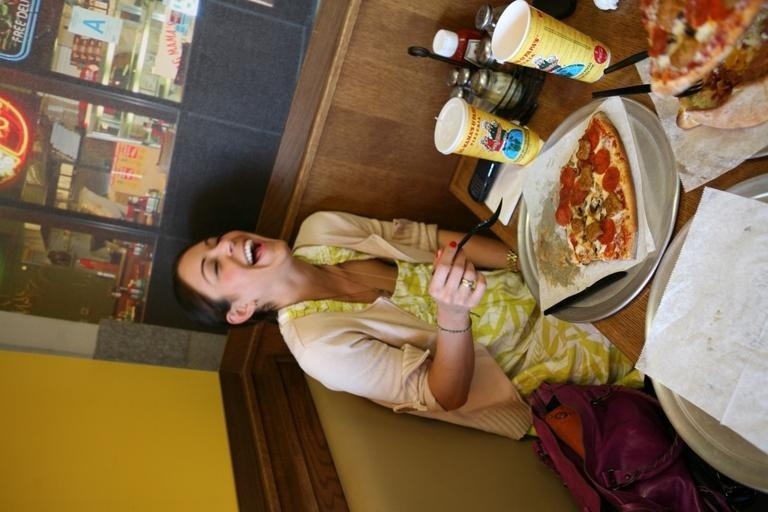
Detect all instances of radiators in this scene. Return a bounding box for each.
[450,1,767,371]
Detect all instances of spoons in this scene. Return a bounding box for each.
[431,196,504,261]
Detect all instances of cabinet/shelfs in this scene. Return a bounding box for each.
[85,0,173,147]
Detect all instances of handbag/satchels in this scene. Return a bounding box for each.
[522,376,733,511]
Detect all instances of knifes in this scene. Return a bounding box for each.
[543,271,629,316]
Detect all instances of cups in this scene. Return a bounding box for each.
[490,1,612,84]
[433,96,546,167]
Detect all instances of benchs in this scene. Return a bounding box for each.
[305,375,583,511]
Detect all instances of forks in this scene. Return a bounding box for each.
[591,77,704,100]
